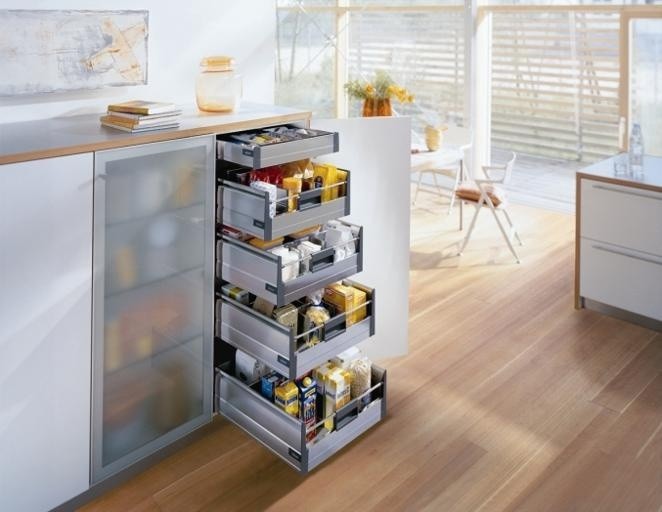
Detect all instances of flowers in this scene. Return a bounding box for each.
[342,68,415,104]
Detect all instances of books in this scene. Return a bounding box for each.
[98,99,183,134]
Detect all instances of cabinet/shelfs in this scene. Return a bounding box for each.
[573,151,662,333]
[1,98,411,512]
[362,99,393,117]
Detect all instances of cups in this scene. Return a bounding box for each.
[613,154,628,175]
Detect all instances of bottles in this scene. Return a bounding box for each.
[193,54,245,113]
[628,122,645,174]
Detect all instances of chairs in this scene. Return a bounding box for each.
[411,128,524,264]
